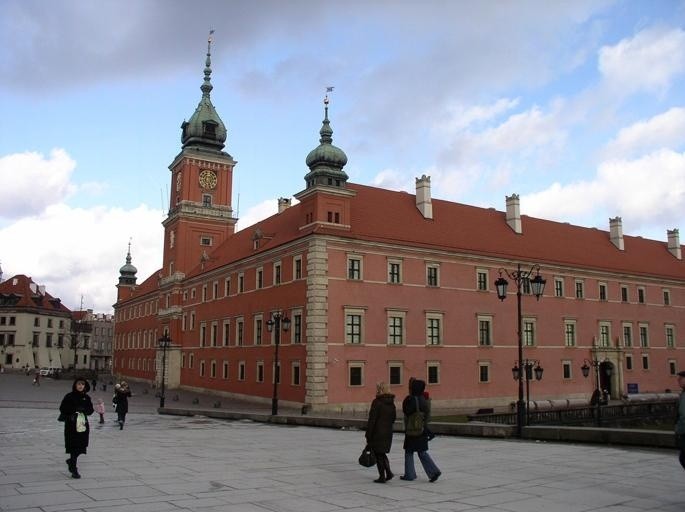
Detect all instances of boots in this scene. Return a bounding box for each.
[372,471,386,483]
[386,466,396,480]
[118,420,124,430]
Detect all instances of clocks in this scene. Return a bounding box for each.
[198,168,220,190]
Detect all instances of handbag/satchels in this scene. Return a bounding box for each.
[57,412,66,422]
[358,446,378,468]
[406,410,425,435]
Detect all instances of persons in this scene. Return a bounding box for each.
[57,377,93,479]
[92,379,96,391]
[112,383,122,407]
[96,398,105,423]
[408,376,436,440]
[400,379,441,482]
[112,379,131,430]
[677,370,685,469]
[366,380,395,483]
[31,372,39,387]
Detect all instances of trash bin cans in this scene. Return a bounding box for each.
[302,405,307,414]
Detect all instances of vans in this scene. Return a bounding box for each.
[39,366,62,377]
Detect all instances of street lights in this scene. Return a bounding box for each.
[158,327,173,408]
[510,358,544,426]
[265,306,291,415]
[494,263,549,426]
[582,355,613,425]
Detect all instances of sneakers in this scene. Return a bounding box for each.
[399,474,413,480]
[429,470,441,482]
[71,468,81,478]
[65,457,72,474]
